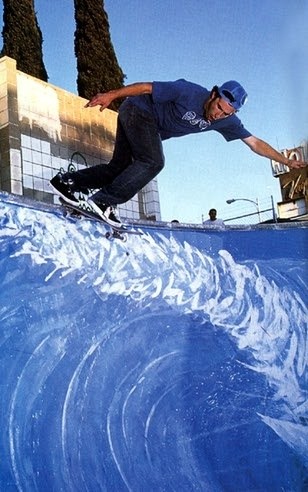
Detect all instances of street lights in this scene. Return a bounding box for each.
[226,198,260,224]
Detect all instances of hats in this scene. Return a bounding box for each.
[217,80,249,113]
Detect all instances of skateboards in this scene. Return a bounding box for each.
[59,197,143,242]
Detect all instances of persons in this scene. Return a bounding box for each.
[48,78,307,231]
[203,208,226,229]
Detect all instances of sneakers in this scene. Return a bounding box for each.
[87,198,122,229]
[46,172,81,207]
